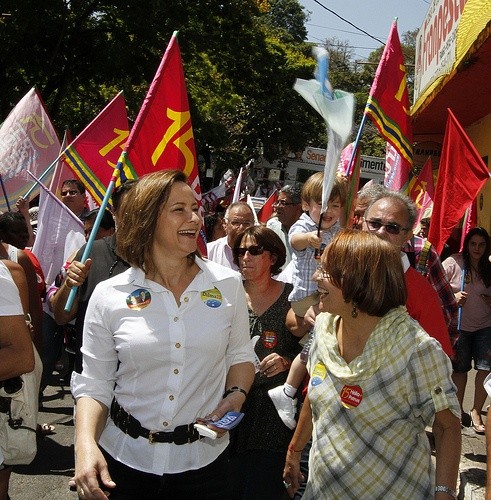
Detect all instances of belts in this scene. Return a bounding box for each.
[111,398,208,445]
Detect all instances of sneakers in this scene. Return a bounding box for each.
[268,382,301,431]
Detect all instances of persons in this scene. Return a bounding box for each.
[0,179,490,500]
[282,229,461,500]
[68,169,255,500]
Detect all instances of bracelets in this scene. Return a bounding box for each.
[287,447,305,453]
[222,386,247,398]
[435,486,456,499]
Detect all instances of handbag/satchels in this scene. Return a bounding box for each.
[0,341,43,466]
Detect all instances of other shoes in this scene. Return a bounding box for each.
[468,408,486,433]
[35,422,55,435]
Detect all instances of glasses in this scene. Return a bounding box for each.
[317,263,344,279]
[364,219,408,235]
[232,246,264,258]
[60,190,77,196]
[276,200,296,206]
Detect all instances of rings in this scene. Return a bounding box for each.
[283,481,292,488]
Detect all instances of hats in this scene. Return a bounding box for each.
[28,206,39,225]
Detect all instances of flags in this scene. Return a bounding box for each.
[0,16,491,324]
